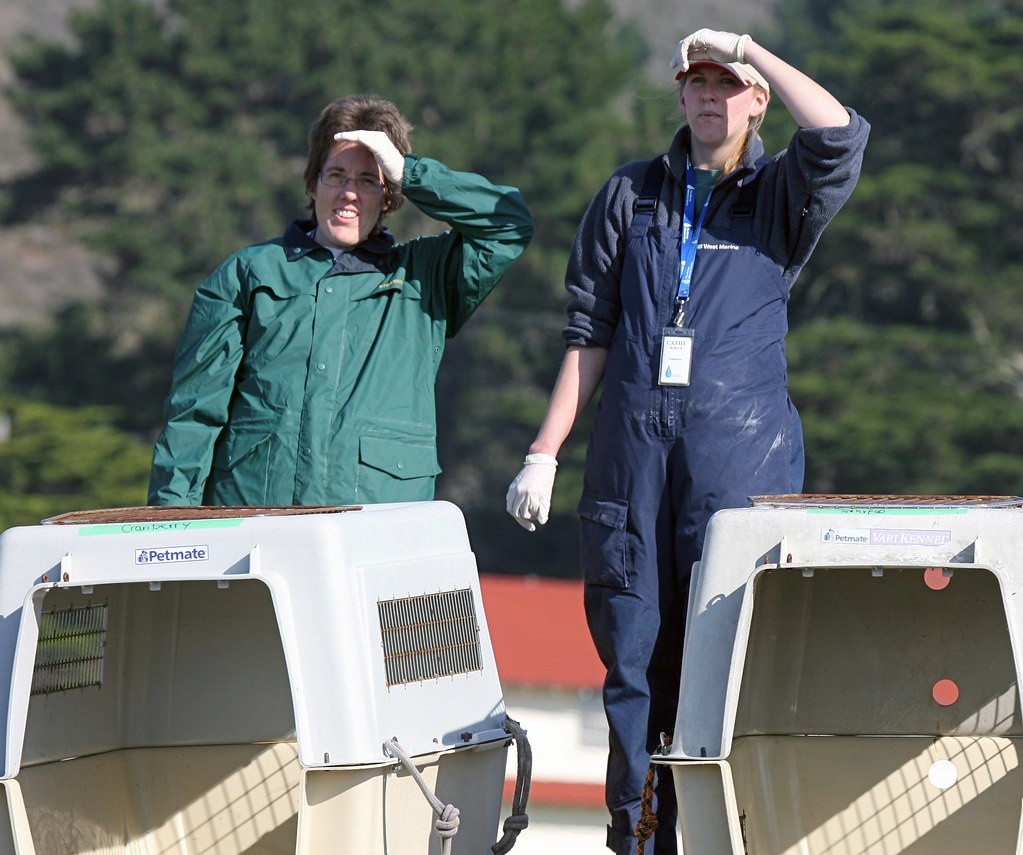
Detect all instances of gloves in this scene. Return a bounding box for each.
[668,27,752,73]
[335,128,403,187]
[508,454,558,532]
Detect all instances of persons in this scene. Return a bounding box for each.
[145,92,536,507]
[504,27,873,855]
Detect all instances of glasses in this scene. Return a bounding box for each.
[319,168,391,193]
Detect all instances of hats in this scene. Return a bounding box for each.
[674,55,771,90]
